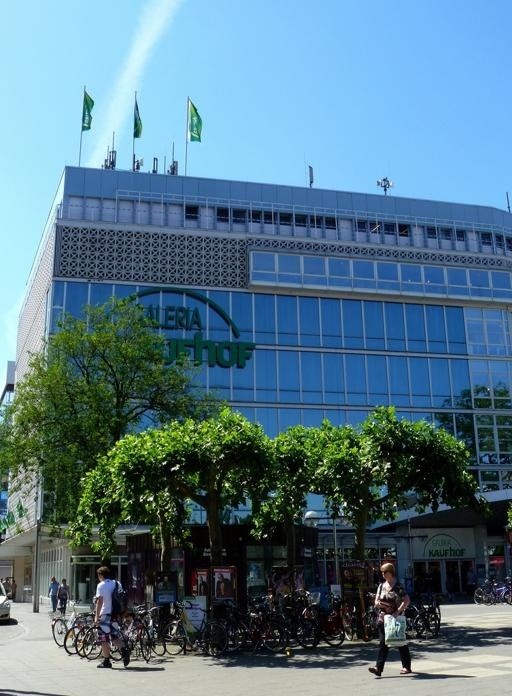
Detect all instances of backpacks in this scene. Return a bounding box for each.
[112,580,127,615]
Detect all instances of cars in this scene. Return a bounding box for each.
[0,582,13,621]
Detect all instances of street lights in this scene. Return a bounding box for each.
[304,511,319,587]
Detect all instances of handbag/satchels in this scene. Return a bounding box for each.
[384,614,408,647]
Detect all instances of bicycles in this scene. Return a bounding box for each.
[163,593,353,656]
[473,577,512,606]
[361,592,441,642]
[51,600,166,662]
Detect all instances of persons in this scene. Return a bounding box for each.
[57,578,71,616]
[466,567,476,602]
[11,580,17,602]
[47,576,61,613]
[95,566,131,669]
[341,567,361,584]
[3,578,11,595]
[368,563,412,678]
[215,573,233,598]
[198,575,209,597]
[144,574,155,609]
[158,577,174,591]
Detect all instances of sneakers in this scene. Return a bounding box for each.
[122,649,130,666]
[400,667,412,674]
[369,668,381,676]
[97,660,112,668]
